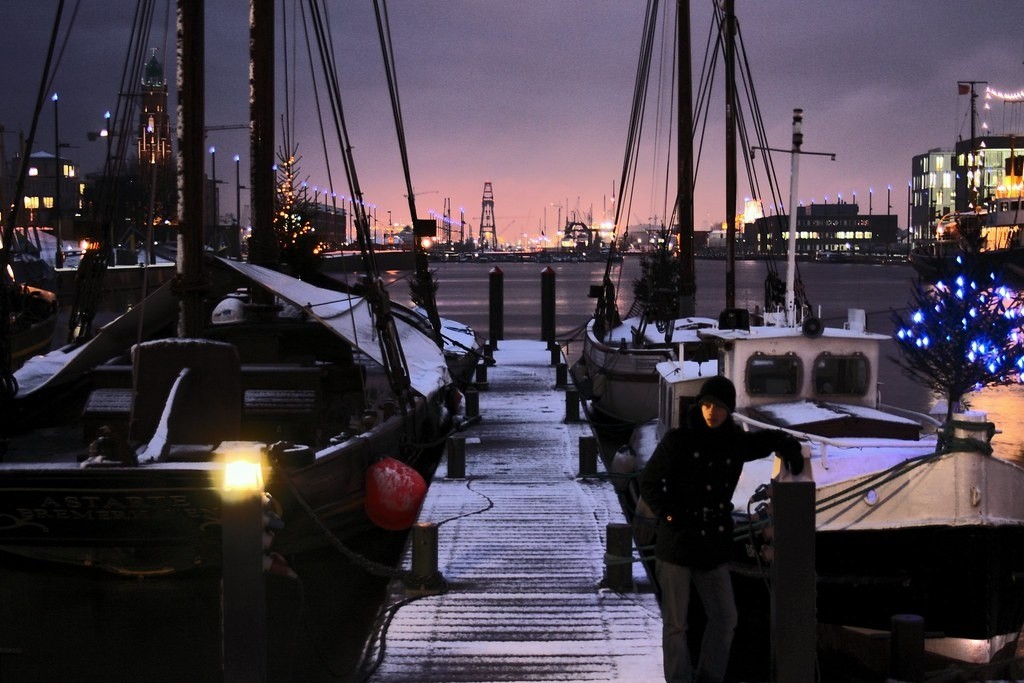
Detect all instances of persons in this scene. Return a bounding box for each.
[638,376,804,683]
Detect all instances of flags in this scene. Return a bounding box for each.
[959,84,969,96]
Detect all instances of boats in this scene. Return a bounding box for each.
[621,106,1024,673]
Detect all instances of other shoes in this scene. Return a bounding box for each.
[696,671,715,683]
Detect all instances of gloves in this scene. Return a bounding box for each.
[672,504,696,530]
[778,434,804,476]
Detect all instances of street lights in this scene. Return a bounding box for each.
[342,195,345,242]
[374,205,377,245]
[303,183,308,220]
[387,211,392,249]
[907,181,911,256]
[769,187,872,215]
[209,144,218,256]
[233,155,245,262]
[368,204,371,229]
[103,109,113,182]
[887,184,891,216]
[324,191,328,236]
[51,92,66,270]
[332,193,337,241]
[273,165,278,212]
[349,197,352,243]
[314,187,317,227]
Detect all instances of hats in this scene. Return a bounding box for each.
[698,376,736,414]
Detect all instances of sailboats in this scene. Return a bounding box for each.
[0,0,483,683]
[581,0,822,429]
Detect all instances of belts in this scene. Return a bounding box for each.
[686,506,720,522]
